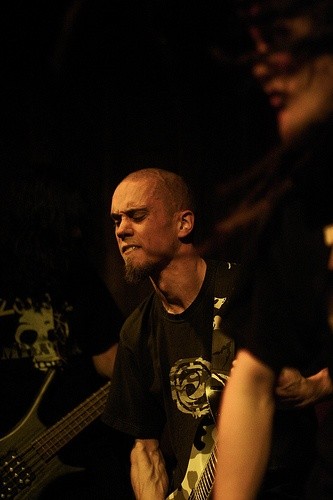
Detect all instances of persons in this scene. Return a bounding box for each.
[105,170,331,500]
[211,0,333,499]
[1,181,124,499]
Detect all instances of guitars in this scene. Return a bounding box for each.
[0,369,113,500]
[162,413,219,500]
